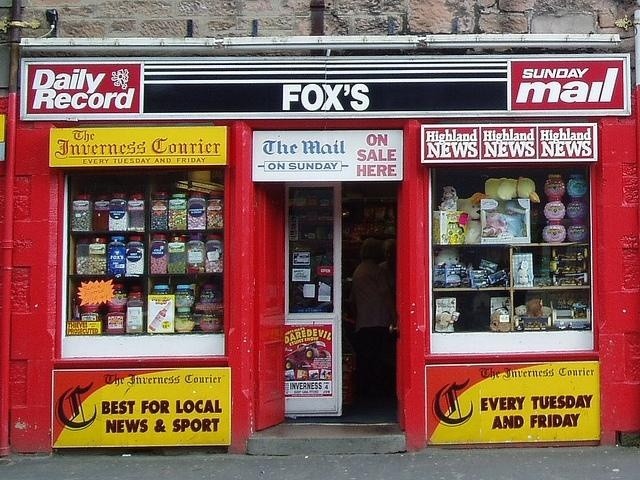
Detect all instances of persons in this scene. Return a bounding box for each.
[343,238,396,400]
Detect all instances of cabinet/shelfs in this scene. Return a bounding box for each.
[62,173,224,360]
[430,168,595,355]
[289,197,398,285]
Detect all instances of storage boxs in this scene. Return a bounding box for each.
[432,208,468,245]
[478,196,533,246]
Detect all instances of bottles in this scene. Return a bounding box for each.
[543,173,587,243]
[66,191,222,335]
